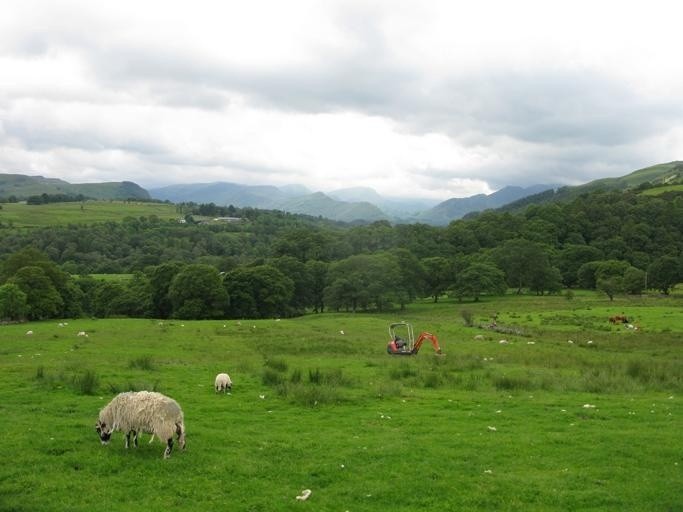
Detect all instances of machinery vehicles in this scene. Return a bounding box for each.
[387,322,446,362]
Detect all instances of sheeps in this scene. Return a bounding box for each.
[214,373,233,394]
[95,390,187,459]
